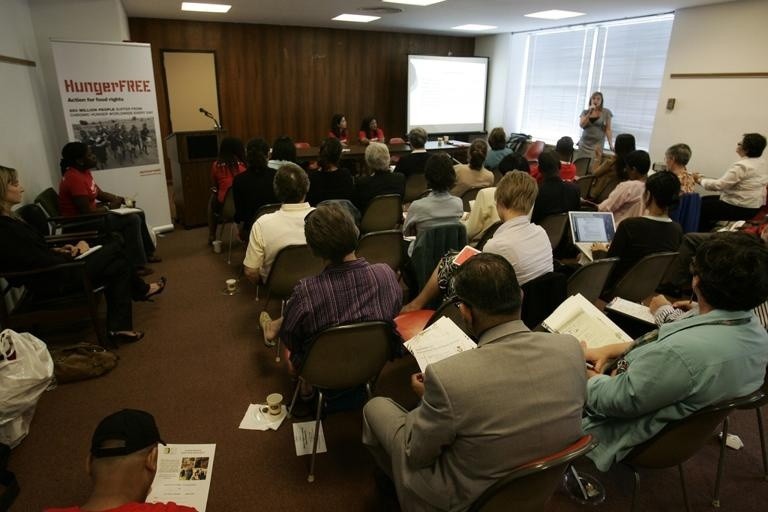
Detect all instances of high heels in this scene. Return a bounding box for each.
[142,276,167,303]
[107,331,146,344]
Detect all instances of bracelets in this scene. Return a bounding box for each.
[114,194,118,200]
[104,206,109,212]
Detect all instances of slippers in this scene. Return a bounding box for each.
[260,312,276,347]
[299,386,315,400]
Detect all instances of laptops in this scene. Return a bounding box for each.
[568,210,618,263]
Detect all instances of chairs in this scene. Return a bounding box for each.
[1,187,138,349]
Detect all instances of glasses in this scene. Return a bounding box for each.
[738,141,744,148]
[455,301,462,308]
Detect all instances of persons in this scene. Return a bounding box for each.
[183,457,206,480]
[0,165,167,348]
[333,251,589,511]
[326,113,350,147]
[581,232,768,473]
[357,116,385,147]
[211,127,768,420]
[80,121,150,170]
[57,142,162,277]
[576,91,614,169]
[43,408,198,512]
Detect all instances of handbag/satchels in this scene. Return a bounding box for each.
[507,133,532,156]
[51,343,119,382]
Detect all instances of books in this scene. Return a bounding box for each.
[108,207,143,215]
[73,245,103,261]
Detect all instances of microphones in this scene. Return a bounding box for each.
[198,107,211,117]
[590,102,597,114]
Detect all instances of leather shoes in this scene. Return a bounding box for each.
[147,254,161,263]
[136,267,153,276]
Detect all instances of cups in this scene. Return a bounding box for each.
[443,135,448,144]
[267,392,283,419]
[436,137,443,146]
[225,278,236,296]
[212,241,221,254]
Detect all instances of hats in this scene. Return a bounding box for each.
[90,408,165,457]
